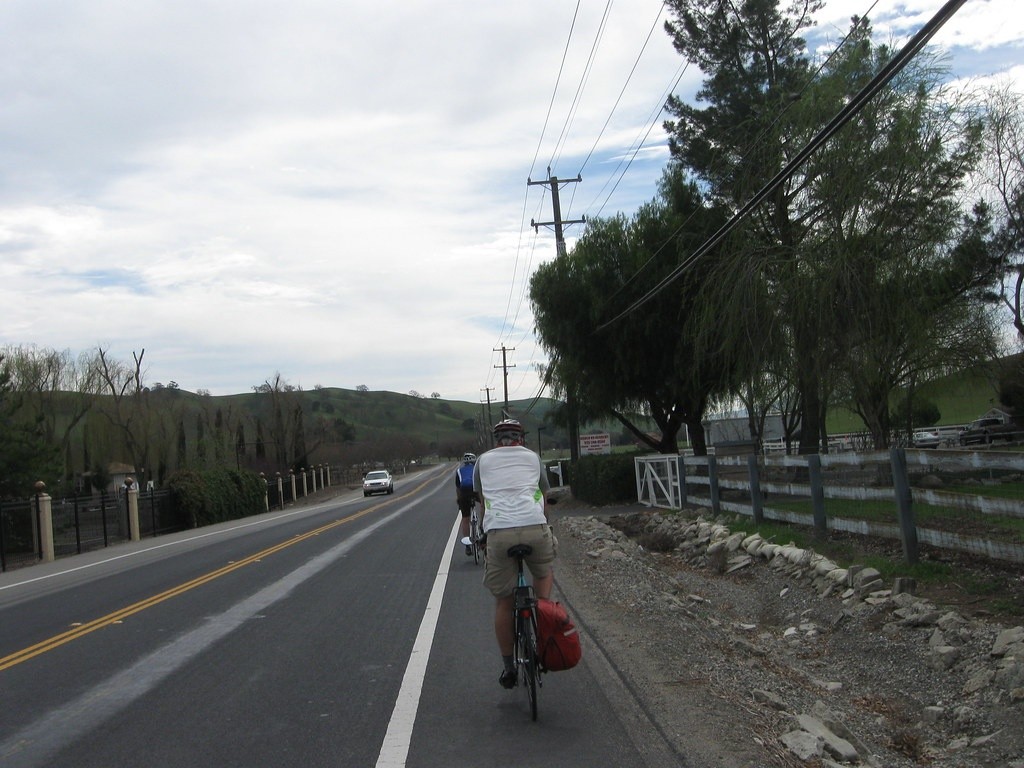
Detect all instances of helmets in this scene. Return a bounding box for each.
[463,453,477,464]
[494,419,522,433]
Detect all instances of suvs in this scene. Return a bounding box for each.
[362,469,394,497]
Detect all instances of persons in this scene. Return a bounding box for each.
[472,419,554,685]
[455,453,481,554]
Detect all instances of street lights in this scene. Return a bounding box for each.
[537,426,547,457]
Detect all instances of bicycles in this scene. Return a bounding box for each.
[472,521,554,721]
[456,493,484,566]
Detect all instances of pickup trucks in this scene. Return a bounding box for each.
[959,417,1018,446]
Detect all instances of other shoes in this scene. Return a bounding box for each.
[466,545,471,556]
[499,670,517,689]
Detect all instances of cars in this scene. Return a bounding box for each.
[906,431,941,449]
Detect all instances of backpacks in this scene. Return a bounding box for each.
[535,597,582,674]
